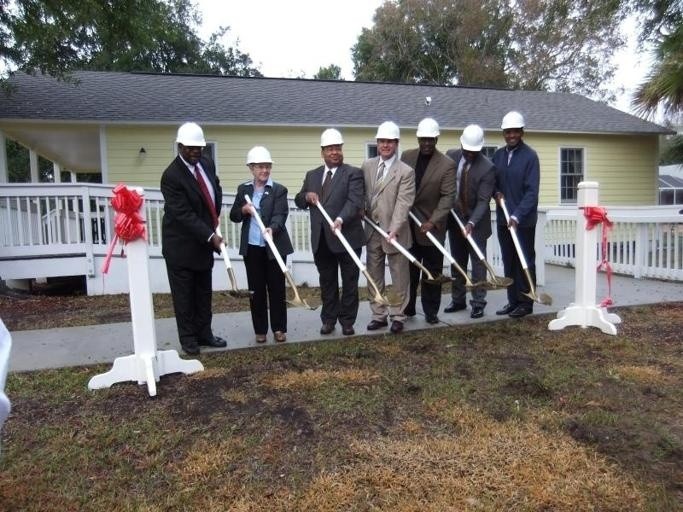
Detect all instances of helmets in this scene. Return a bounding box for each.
[320,128,344,147]
[246,146,272,165]
[416,118,440,138]
[459,124,484,152]
[375,121,400,139]
[175,122,206,147]
[501,111,524,129]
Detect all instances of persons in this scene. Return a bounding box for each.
[230,146,294,342]
[361,121,416,332]
[295,129,365,335]
[446,124,495,318]
[491,112,539,317]
[160,123,227,355]
[400,118,456,323]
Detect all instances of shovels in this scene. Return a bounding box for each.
[215,224,255,298]
[315,200,406,307]
[450,208,514,287]
[499,197,553,305]
[244,194,318,311]
[362,214,456,285]
[409,211,494,290]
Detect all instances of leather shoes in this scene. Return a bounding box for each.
[343,325,354,335]
[425,302,484,323]
[391,321,403,332]
[181,341,199,355]
[321,324,334,334]
[200,336,227,347]
[367,320,388,330]
[274,331,286,341]
[508,304,533,318]
[255,334,266,342]
[495,302,518,314]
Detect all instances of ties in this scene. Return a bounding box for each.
[194,165,219,224]
[371,162,384,224]
[322,171,332,204]
[459,161,468,218]
